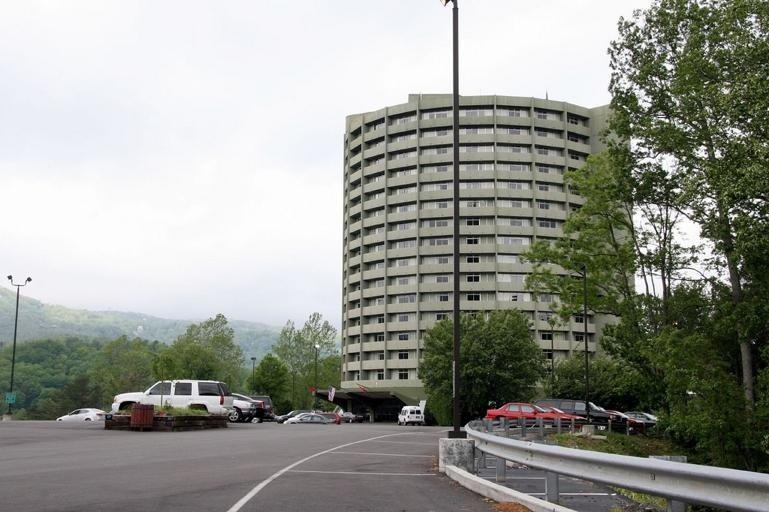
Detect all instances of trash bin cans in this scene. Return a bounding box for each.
[130,403,154,431]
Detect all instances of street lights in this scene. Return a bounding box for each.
[6,275,32,415]
[440,0,467,438]
[313,341,320,410]
[251,357,257,391]
[562,255,589,425]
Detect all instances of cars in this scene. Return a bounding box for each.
[56,407,108,422]
[487,398,661,436]
[111,378,233,415]
[229,392,363,425]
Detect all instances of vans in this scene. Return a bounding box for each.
[398,405,425,426]
